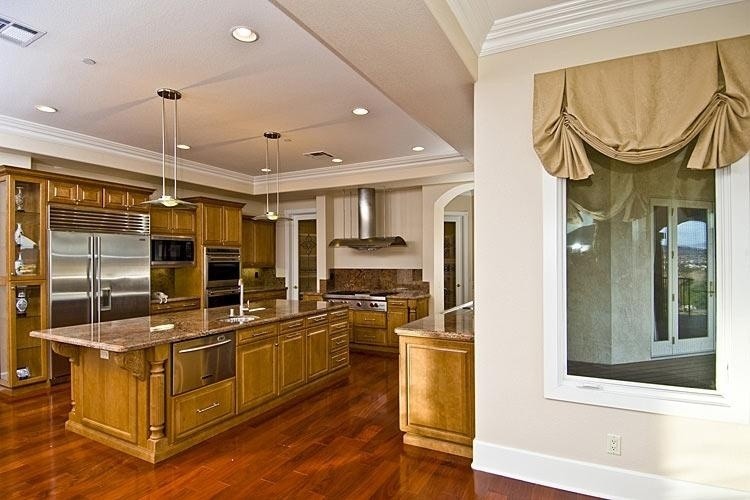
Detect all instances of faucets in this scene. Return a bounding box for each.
[239,278,250,316]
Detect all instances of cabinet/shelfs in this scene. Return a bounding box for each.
[103,188,150,213]
[392,328,476,460]
[0,165,47,401]
[387,300,428,349]
[237,305,351,414]
[67,374,236,443]
[150,194,193,236]
[347,308,388,346]
[200,203,241,248]
[241,216,276,268]
[47,180,103,212]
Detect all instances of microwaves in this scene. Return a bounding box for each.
[204,246,242,308]
[151,234,196,265]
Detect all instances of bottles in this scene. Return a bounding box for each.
[15,188,25,211]
[14,222,24,245]
[229,309,235,317]
[15,252,24,275]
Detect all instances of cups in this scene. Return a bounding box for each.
[16,368,29,378]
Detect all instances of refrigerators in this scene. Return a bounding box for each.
[47,203,151,386]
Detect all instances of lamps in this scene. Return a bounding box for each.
[253,133,292,223]
[139,89,196,209]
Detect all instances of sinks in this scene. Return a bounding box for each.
[217,315,261,326]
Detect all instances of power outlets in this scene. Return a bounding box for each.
[607,432,621,456]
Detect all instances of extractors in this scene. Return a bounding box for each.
[328,188,409,256]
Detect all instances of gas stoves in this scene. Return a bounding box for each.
[322,290,400,313]
